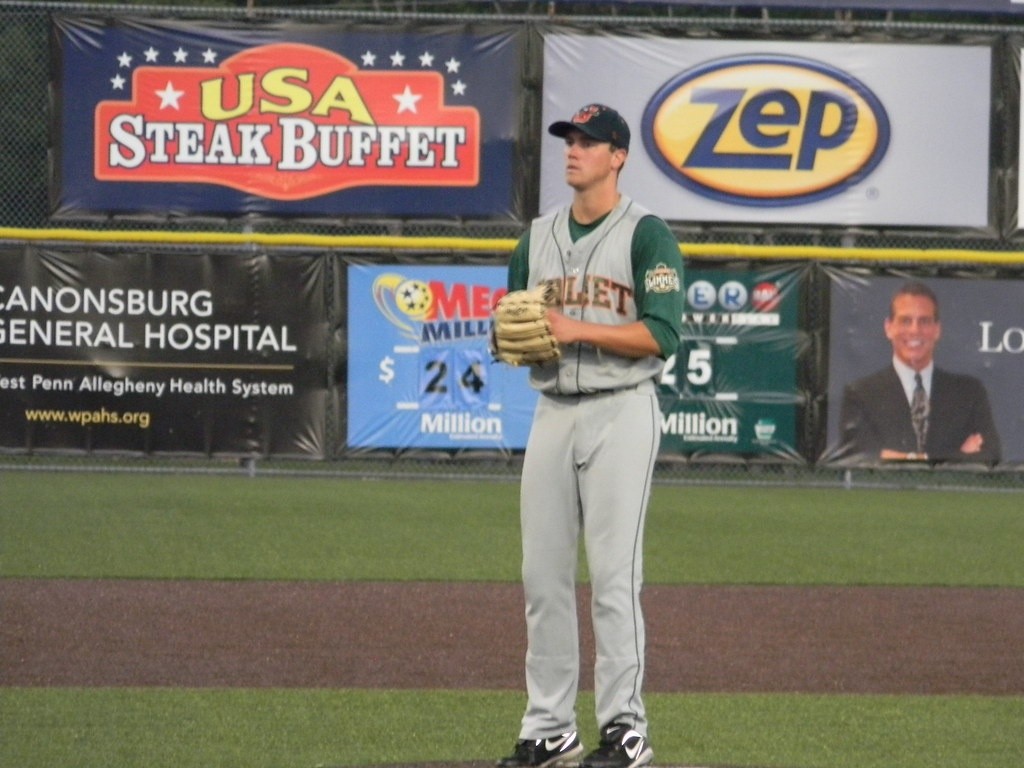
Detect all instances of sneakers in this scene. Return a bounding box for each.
[581,724,654,767]
[497,730,581,767]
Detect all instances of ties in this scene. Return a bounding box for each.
[911,373,930,446]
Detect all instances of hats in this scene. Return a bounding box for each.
[549,105,631,151]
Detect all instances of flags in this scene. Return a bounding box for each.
[48,10,527,227]
[524,20,1010,243]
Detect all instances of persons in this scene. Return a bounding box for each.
[487,106,686,767]
[840,280,1004,473]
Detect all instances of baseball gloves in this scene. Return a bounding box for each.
[493,286,561,364]
